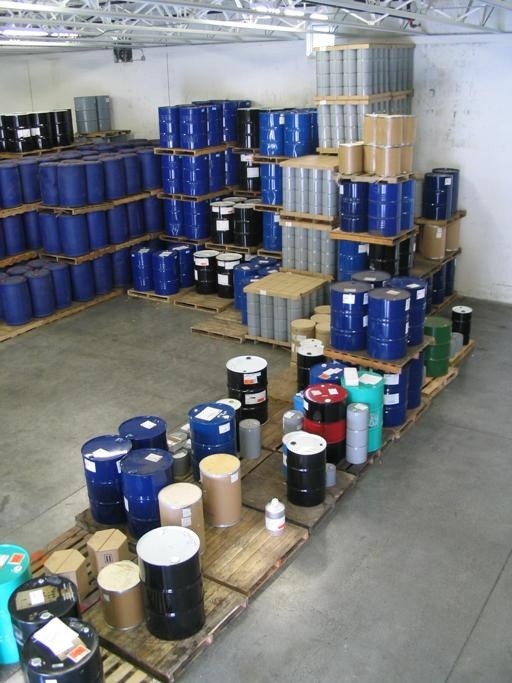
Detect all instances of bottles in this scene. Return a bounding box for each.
[263,497,286,536]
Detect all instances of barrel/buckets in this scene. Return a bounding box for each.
[1,95,471,683]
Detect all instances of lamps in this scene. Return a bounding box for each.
[302,10,336,57]
[83,30,135,63]
